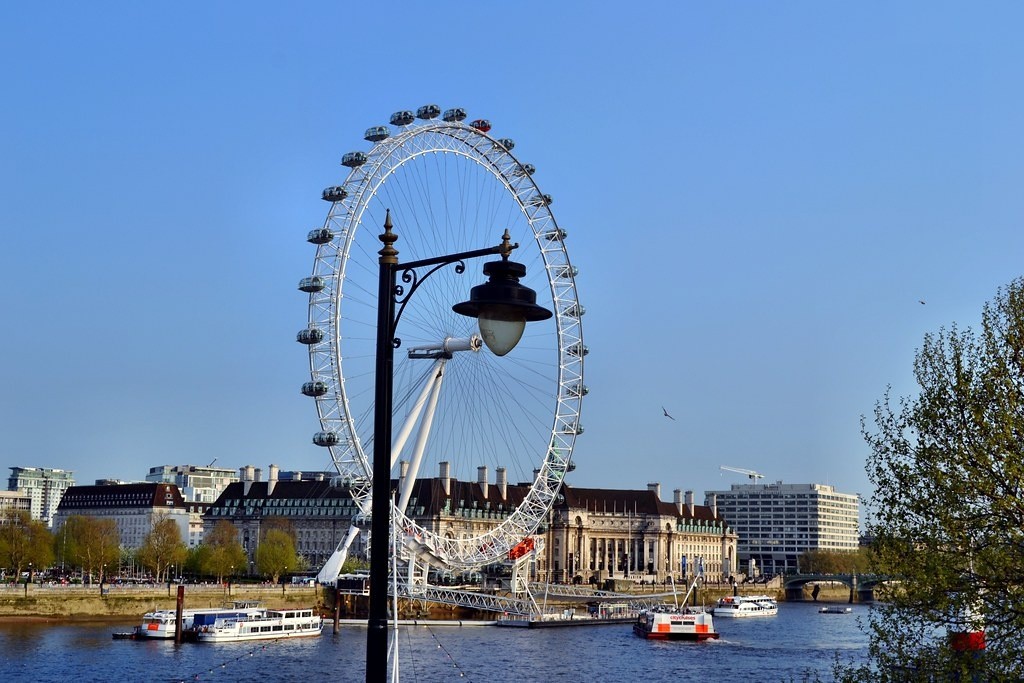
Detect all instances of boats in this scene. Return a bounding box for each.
[183,600,327,642]
[714,594,779,619]
[818,605,854,615]
[111,624,142,640]
[140,603,232,641]
[632,571,721,642]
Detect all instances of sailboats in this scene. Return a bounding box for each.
[943,537,987,653]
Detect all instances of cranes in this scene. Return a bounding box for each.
[718,464,766,485]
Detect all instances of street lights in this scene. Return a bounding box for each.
[282,566,289,595]
[364,205,556,683]
[316,564,319,584]
[168,564,175,597]
[24,562,33,596]
[228,564,235,597]
[100,562,108,597]
